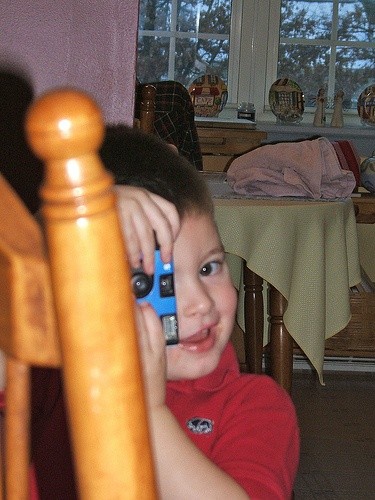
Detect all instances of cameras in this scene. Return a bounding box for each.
[129,244,179,347]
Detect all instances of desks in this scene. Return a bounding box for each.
[213,198,354,395]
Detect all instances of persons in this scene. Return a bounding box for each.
[29,120,300,499]
